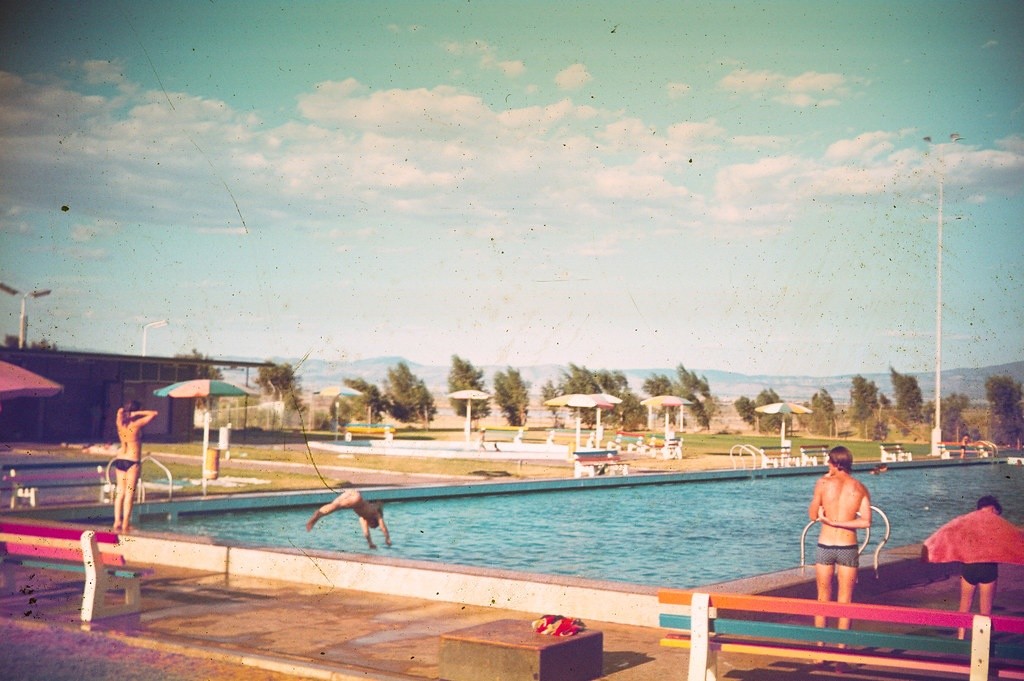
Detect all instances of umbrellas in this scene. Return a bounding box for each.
[319,386,364,439]
[542,395,695,450]
[0,360,64,401]
[446,390,491,442]
[754,403,814,447]
[153,379,260,494]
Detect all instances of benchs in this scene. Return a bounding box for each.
[880,443,913,463]
[614,432,684,460]
[938,443,991,461]
[760,446,800,468]
[342,426,395,441]
[0,523,154,625]
[572,450,630,479]
[656,588,1024,681]
[0,455,118,510]
[799,445,830,466]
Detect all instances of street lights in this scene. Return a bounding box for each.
[0,281,52,350]
[922,131,963,456]
[141,318,169,357]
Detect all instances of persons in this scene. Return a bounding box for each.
[807,447,872,674]
[923,496,1023,640]
[960,436,969,459]
[111,401,157,536]
[305,490,390,551]
